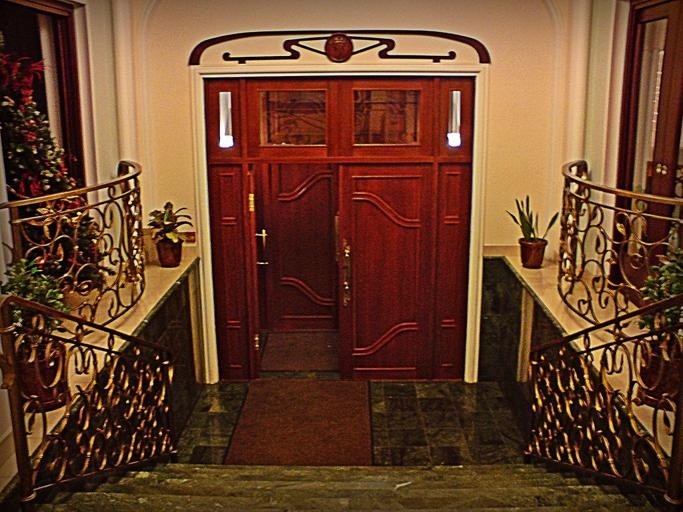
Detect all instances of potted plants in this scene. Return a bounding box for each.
[0,259,69,412]
[638,249,683,412]
[148,202,193,267]
[506,196,557,270]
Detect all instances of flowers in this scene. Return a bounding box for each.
[0,59,116,291]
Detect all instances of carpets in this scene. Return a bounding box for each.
[222,380,375,465]
[260,332,340,372]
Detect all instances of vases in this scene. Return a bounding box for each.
[61,288,99,309]
